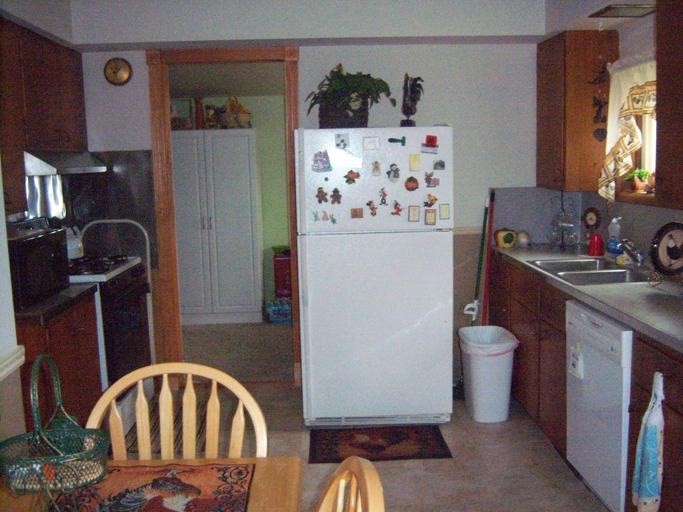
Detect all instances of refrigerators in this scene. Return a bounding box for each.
[292,127,456,433]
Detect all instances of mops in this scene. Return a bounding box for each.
[452,207,488,400]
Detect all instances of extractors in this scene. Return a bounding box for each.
[23,150,107,177]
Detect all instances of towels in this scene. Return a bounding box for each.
[629,369,665,510]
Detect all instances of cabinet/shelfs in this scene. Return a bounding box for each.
[625,330,683,511]
[15,289,102,437]
[169,128,265,329]
[654,3,683,210]
[532,29,620,193]
[18,24,88,155]
[0,13,30,218]
[508,264,567,460]
[486,253,508,333]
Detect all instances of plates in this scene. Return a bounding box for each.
[650,222,683,276]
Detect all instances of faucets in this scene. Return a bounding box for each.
[616,239,645,267]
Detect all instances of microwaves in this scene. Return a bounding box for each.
[5,228,72,314]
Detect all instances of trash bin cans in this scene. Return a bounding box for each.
[457,325,520,423]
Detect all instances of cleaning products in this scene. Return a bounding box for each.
[605,216,623,260]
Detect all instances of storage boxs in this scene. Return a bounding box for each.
[271,251,291,296]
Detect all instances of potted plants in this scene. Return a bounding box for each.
[624,169,649,194]
[304,62,397,128]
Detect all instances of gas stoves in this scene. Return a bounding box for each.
[69,254,146,305]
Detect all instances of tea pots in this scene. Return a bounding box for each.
[60,227,86,259]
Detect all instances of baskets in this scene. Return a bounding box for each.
[217,113,250,128]
[0,354,108,493]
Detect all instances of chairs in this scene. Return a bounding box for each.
[313,455,386,512]
[76,360,269,462]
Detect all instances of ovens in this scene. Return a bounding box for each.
[103,284,153,392]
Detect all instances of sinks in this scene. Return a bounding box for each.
[552,270,664,287]
[526,256,643,272]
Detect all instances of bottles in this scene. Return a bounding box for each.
[607,217,623,257]
[587,234,604,257]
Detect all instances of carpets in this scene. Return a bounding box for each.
[304,422,452,464]
[122,390,209,460]
